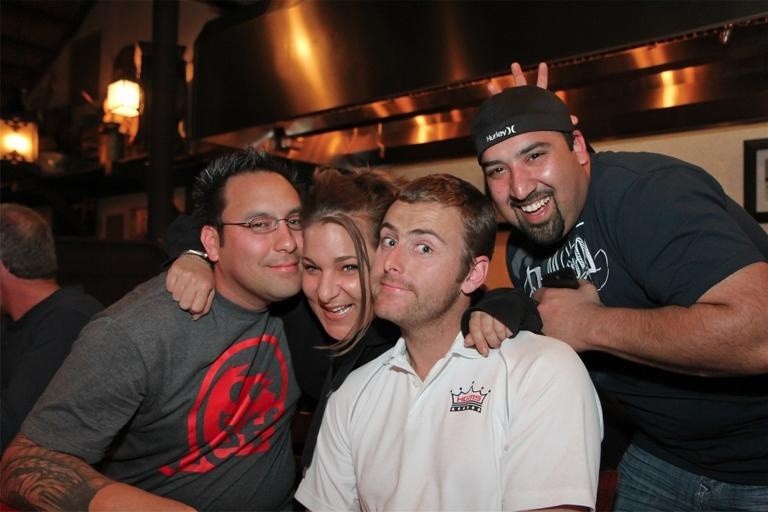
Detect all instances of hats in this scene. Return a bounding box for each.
[470,84,576,164]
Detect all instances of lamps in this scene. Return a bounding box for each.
[106,79,141,118]
[0,102,41,175]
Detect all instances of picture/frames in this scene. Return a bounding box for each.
[742,137,768,224]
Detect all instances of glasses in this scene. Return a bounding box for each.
[220,214,306,232]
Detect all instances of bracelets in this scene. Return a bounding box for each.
[182,249,209,259]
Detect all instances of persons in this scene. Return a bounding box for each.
[162,158,549,512]
[464,84,768,510]
[0,140,308,512]
[289,169,607,511]
[485,60,580,130]
[1,198,108,455]
